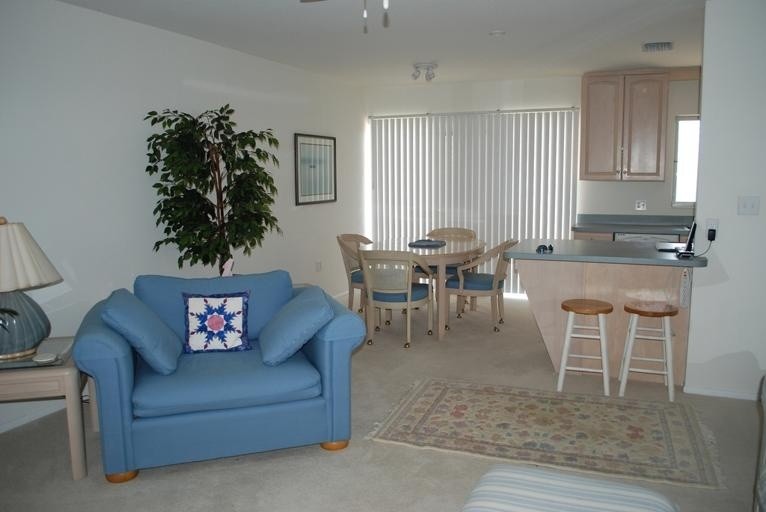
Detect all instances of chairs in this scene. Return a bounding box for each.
[413,228,477,313]
[358,248,434,347]
[445,239,518,332]
[336,233,406,325]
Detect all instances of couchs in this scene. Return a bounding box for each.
[72,270,367,484]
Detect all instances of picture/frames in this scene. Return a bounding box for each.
[294,132,337,205]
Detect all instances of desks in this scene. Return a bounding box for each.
[359,236,486,341]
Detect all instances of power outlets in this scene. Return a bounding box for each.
[737,195,759,215]
[634,199,647,211]
[706,217,720,234]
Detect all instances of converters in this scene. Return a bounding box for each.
[708,229,716,240]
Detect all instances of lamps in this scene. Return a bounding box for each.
[0,216,65,361]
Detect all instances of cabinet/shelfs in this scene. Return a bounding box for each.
[579,68,669,181]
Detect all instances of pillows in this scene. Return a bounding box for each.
[100,288,184,375]
[258,285,336,366]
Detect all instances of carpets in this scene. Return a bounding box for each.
[365,376,728,491]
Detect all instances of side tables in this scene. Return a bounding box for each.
[1,337,97,480]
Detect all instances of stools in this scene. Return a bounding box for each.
[557,299,614,396]
[617,301,679,402]
